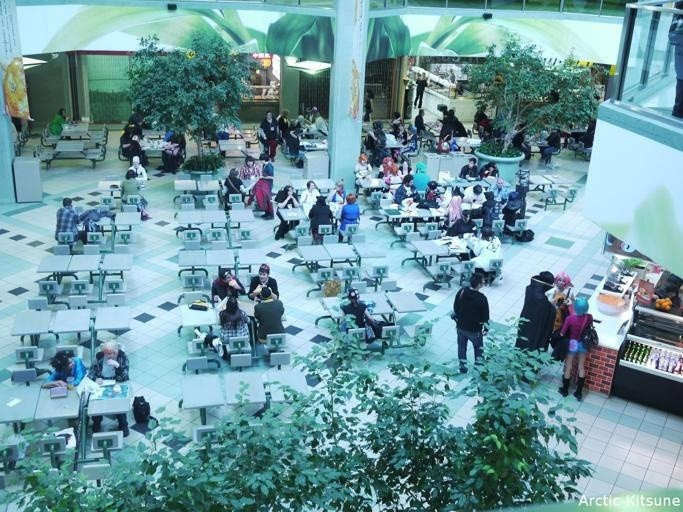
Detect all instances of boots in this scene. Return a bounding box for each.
[558,374,571,397]
[573,376,586,402]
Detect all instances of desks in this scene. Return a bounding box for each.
[0,388,40,433]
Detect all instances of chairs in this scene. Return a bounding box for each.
[7,124,591,487]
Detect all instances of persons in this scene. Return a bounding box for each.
[412,72,428,110]
[452,273,490,373]
[542,271,577,362]
[513,270,557,384]
[212,268,246,306]
[40,349,86,440]
[50,90,531,283]
[559,297,595,400]
[88,339,131,436]
[247,263,279,301]
[218,298,251,343]
[254,287,284,342]
[339,289,375,339]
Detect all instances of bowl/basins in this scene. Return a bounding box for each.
[595,295,626,315]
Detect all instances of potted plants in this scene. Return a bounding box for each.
[457,33,597,183]
[129,23,262,181]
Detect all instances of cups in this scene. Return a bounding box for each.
[66,376,74,389]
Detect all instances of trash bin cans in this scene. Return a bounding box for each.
[424,153,477,181]
[14,157,43,203]
[304,150,330,181]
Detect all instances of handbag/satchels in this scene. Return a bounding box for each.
[581,320,600,348]
